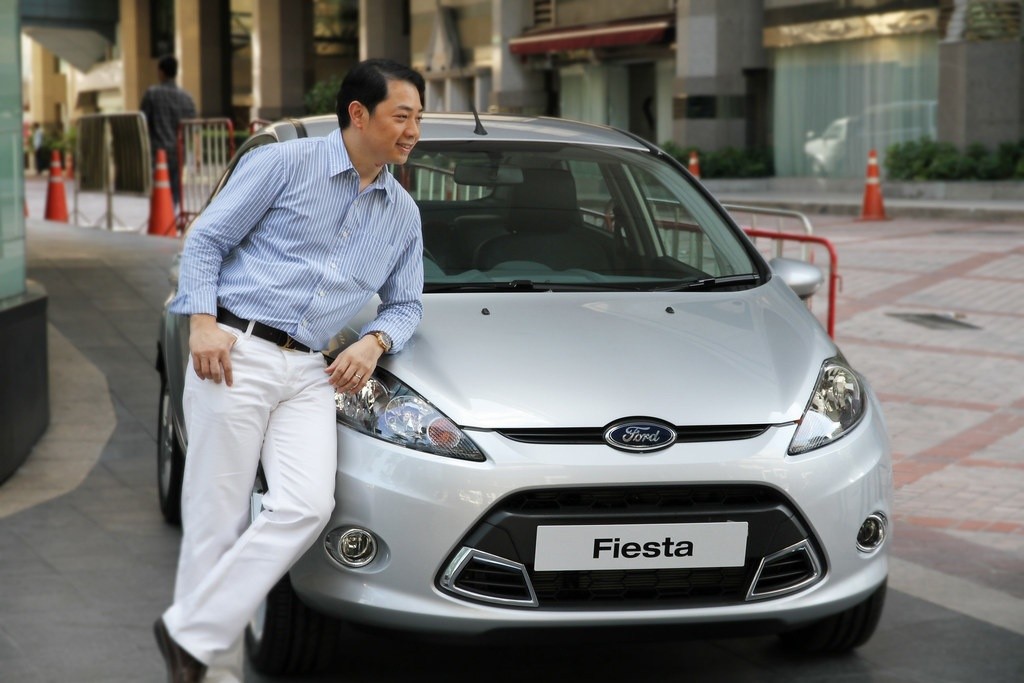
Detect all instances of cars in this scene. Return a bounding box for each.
[154,106,896,683]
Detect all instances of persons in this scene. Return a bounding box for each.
[153,58,426,683]
[141,58,196,207]
[31,123,44,173]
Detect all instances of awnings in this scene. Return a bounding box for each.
[508,12,676,55]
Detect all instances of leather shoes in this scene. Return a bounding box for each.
[154,618,208,683]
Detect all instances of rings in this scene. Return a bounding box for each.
[354,373,361,380]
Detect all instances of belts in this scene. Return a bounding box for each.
[215,306,317,352]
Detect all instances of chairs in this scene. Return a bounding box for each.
[450,214,506,275]
[473,167,614,274]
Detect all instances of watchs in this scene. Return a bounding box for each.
[371,330,392,352]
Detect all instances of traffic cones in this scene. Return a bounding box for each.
[687,150,700,180]
[146,149,177,238]
[852,150,893,223]
[41,151,68,221]
